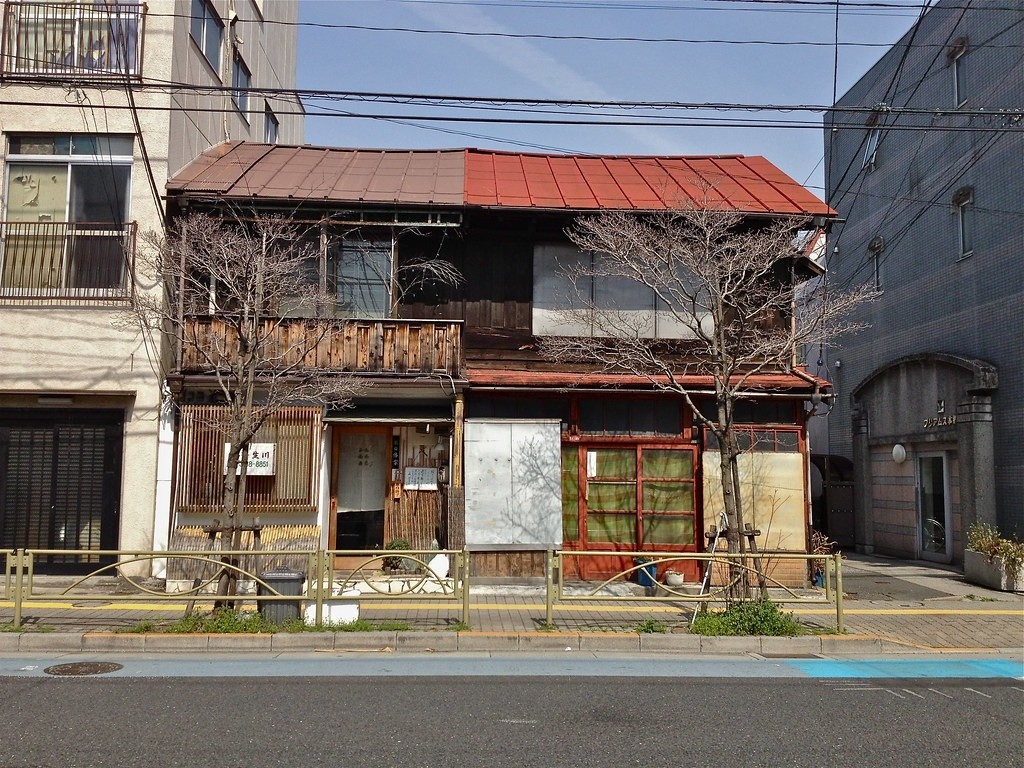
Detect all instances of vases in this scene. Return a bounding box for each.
[665,570,684,585]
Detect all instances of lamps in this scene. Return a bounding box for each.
[892,444,906,463]
[435,435,450,450]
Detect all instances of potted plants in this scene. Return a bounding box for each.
[964,515,1024,592]
[633,550,657,586]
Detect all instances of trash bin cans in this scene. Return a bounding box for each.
[260,565,304,628]
